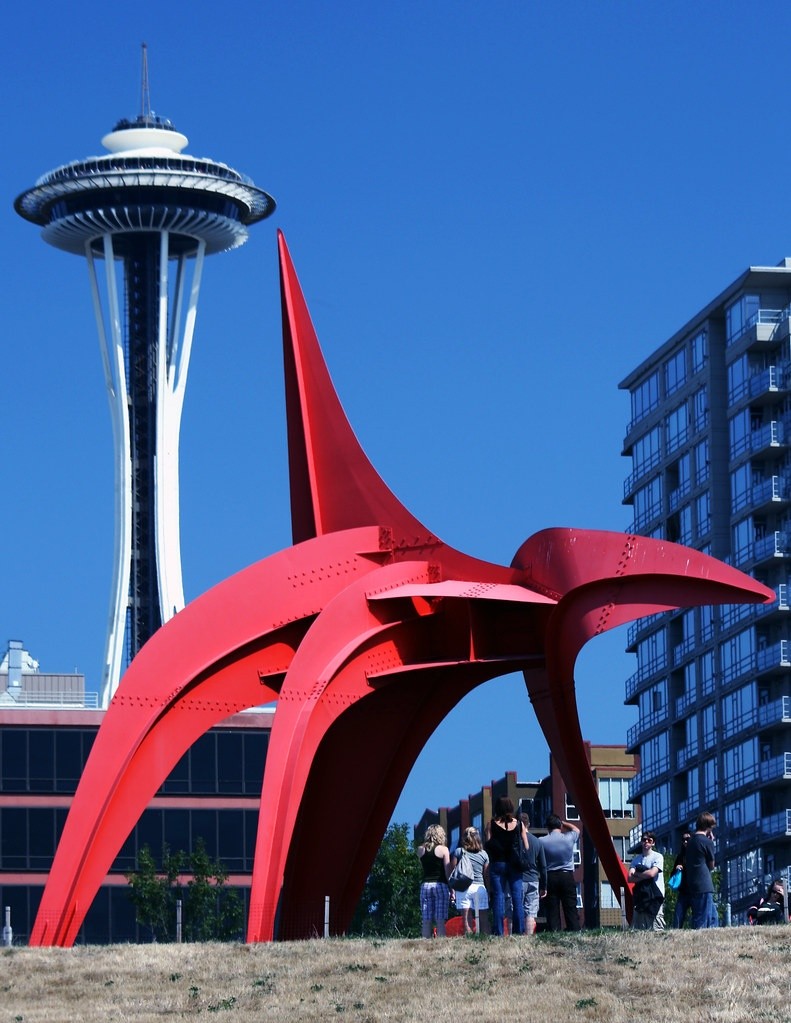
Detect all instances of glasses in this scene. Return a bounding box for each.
[681,837,691,842]
[771,889,781,895]
[642,837,653,843]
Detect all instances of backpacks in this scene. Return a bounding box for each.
[756,900,784,925]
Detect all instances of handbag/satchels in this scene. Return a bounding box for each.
[509,820,530,872]
[446,847,475,892]
[666,870,683,891]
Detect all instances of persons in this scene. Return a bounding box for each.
[670,812,720,929]
[452,827,489,936]
[481,796,529,935]
[627,830,665,932]
[416,824,455,938]
[748,879,791,926]
[503,813,581,935]
